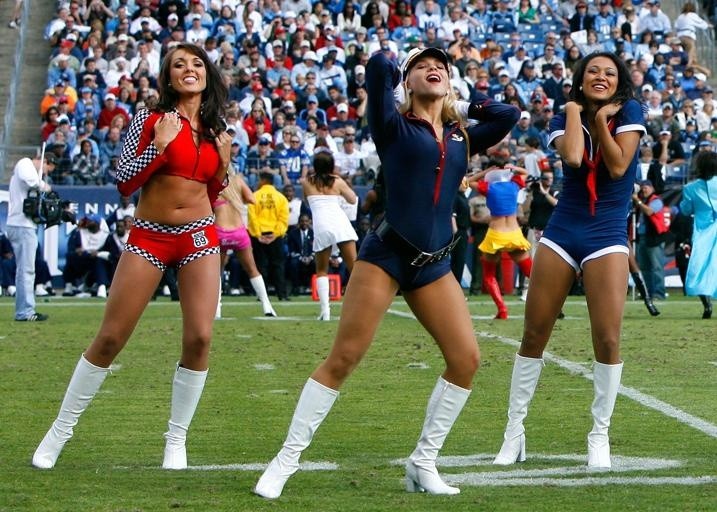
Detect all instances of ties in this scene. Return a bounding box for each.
[303,232,308,255]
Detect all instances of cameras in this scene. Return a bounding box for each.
[525,174,547,191]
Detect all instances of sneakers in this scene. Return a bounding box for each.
[15,313,49,321]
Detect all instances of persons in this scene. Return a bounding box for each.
[28,42,234,471]
[0,0,717,323]
[249,46,521,501]
[491,53,648,473]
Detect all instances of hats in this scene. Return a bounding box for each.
[85,214,101,225]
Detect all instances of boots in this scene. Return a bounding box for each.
[215,276,221,319]
[163,361,209,471]
[316,275,331,321]
[517,257,566,319]
[587,359,623,473]
[699,295,712,320]
[492,352,545,466]
[32,352,111,469]
[253,378,340,498]
[631,270,660,316]
[404,376,473,494]
[250,274,277,316]
[482,258,507,320]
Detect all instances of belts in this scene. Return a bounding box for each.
[376,220,462,268]
